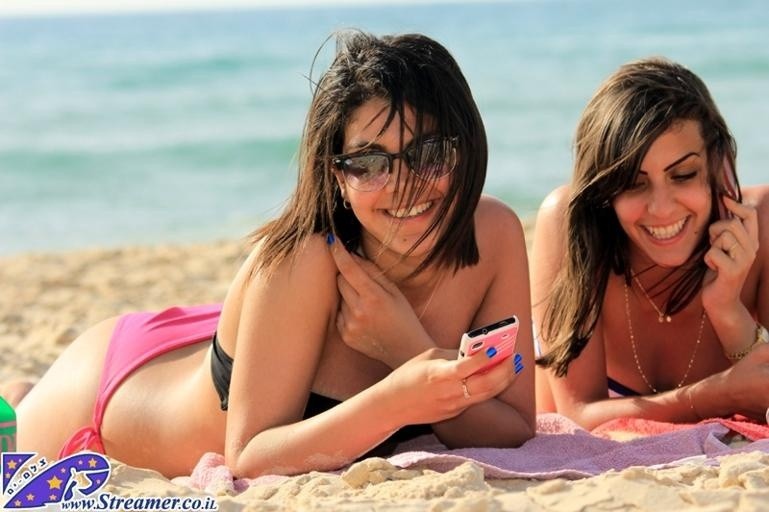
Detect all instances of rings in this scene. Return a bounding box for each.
[463,379,470,400]
[728,241,739,251]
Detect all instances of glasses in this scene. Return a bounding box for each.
[332,134,460,192]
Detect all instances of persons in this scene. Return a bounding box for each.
[9,28,536,484]
[530,57,769,432]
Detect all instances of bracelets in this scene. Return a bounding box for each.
[687,385,700,419]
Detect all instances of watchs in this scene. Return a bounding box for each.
[723,322,769,364]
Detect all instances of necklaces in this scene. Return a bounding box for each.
[623,276,707,394]
[630,269,672,324]
[418,271,444,321]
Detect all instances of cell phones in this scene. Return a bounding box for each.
[458,314,521,371]
